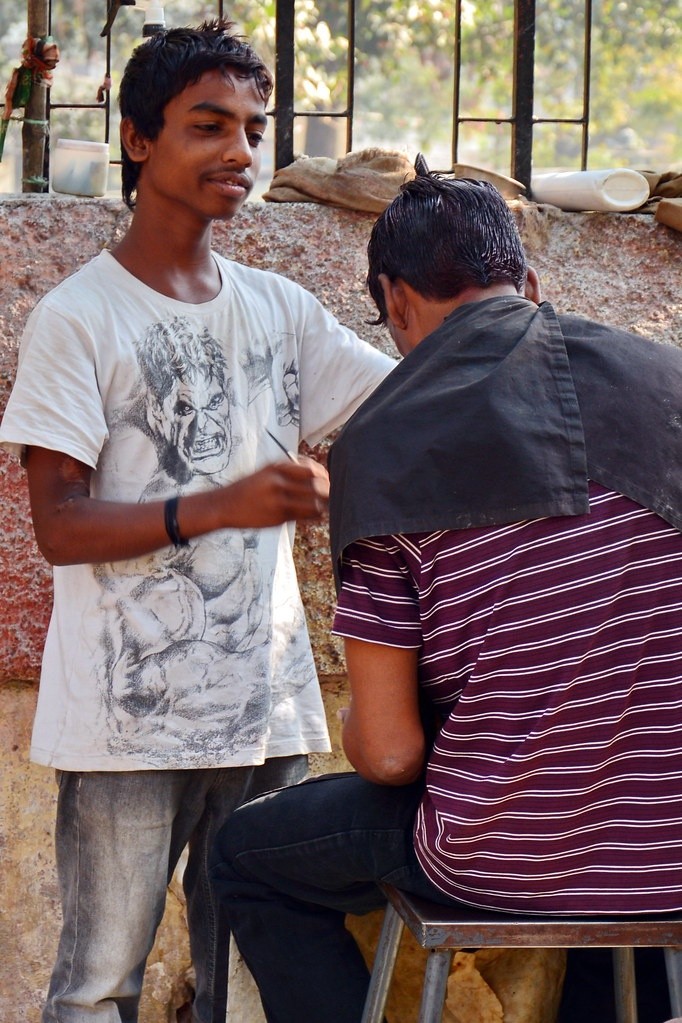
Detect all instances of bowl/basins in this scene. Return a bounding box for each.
[451,163,526,201]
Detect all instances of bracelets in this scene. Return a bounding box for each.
[164,496,189,548]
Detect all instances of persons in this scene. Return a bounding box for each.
[207,153,682,1023]
[0,11,401,1023]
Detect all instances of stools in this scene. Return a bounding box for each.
[353,887,682,1023]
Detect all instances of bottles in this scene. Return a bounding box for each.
[530,168,650,213]
[51,138,109,198]
